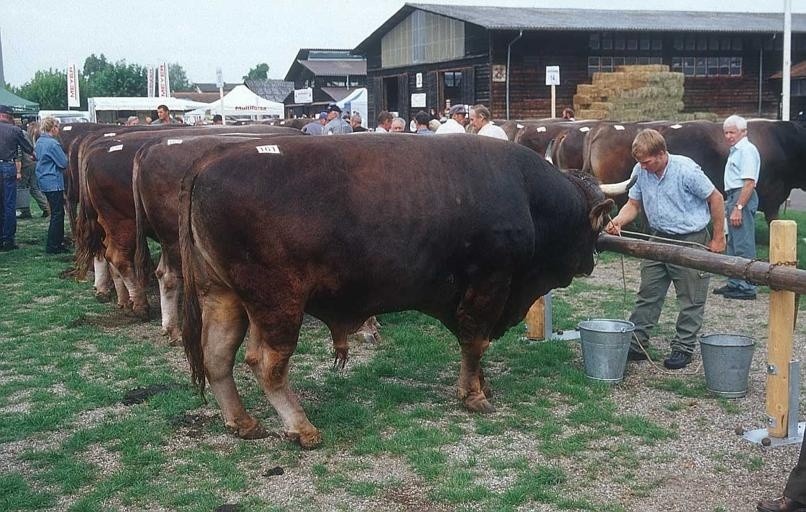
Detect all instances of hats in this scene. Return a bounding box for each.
[0,105,12,114]
[319,111,327,119]
[322,105,342,113]
[449,104,469,115]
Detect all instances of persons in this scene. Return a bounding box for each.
[562,108,575,122]
[0,105,36,251]
[121,104,508,141]
[34,117,70,254]
[604,129,724,368]
[16,129,51,218]
[713,116,762,300]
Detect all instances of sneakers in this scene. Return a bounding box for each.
[15,212,32,219]
[664,351,691,369]
[41,210,50,217]
[627,349,648,360]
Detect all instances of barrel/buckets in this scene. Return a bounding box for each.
[577,318,636,381]
[699,332,756,399]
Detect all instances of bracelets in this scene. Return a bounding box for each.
[17,172,21,174]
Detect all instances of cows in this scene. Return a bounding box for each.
[180,133,615,450]
[466,117,677,228]
[54,119,381,346]
[625,121,806,240]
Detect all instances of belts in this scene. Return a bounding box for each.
[0,159,15,163]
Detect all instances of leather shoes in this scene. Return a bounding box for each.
[0,244,19,252]
[724,289,755,299]
[713,285,737,295]
[50,247,69,253]
[62,239,72,244]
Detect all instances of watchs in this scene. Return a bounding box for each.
[735,204,744,210]
